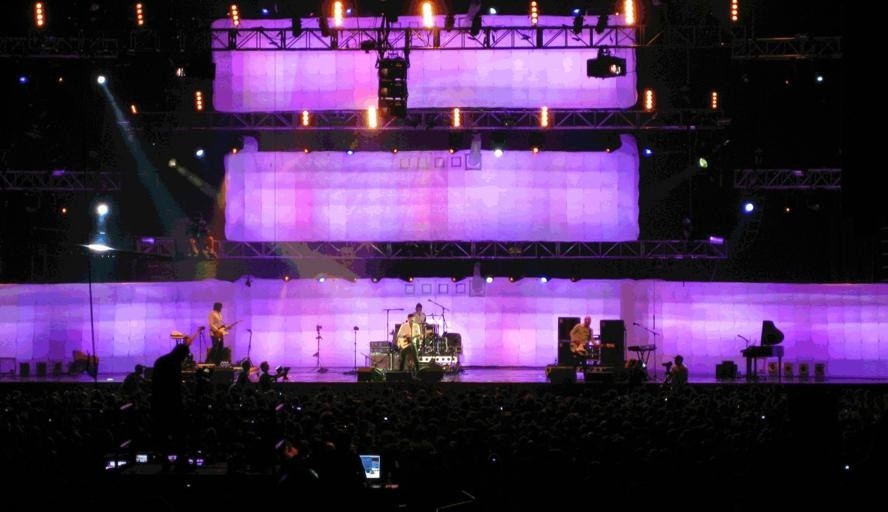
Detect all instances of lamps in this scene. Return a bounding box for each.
[283,274,292,282]
[317,274,326,282]
[450,146,461,153]
[541,276,551,282]
[452,274,468,283]
[372,275,382,282]
[484,275,495,283]
[570,275,581,283]
[606,142,621,152]
[510,275,525,282]
[192,146,204,155]
[305,146,313,152]
[399,275,414,283]
[346,148,355,156]
[86,230,116,254]
[389,147,398,153]
[344,273,357,281]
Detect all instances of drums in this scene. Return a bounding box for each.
[425,333,442,352]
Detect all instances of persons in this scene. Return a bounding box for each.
[569,316,597,381]
[209,303,232,351]
[397,303,426,371]
[1,344,888,512]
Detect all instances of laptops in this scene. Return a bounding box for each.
[358,454,382,483]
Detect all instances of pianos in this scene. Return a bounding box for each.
[170,331,182,338]
[627,345,657,351]
[740,321,784,381]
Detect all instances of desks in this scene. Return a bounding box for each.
[105,450,401,512]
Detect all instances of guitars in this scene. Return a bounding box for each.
[575,343,598,354]
[399,333,420,349]
[214,319,243,341]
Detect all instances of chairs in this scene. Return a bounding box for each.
[435,487,477,512]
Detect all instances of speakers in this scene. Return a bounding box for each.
[416,367,443,381]
[544,366,576,382]
[600,320,627,366]
[557,317,581,364]
[358,366,384,381]
[583,366,620,383]
[385,370,413,380]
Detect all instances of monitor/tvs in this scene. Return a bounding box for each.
[102,452,149,472]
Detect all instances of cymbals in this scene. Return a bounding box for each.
[426,315,439,316]
